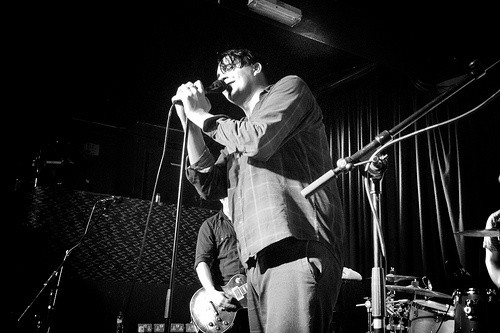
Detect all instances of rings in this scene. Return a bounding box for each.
[187,83,195,89]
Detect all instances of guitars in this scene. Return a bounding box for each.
[190,274,248,333]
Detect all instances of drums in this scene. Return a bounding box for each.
[455,288,499,333]
[409,300,455,333]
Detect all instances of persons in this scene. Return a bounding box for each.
[194,196,248,333]
[171,49,345,333]
[483,175,500,289]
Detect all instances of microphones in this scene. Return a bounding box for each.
[170,78,227,104]
[99,195,124,203]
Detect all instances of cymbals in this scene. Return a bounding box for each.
[454,229,500,238]
[366,275,419,280]
[383,285,453,299]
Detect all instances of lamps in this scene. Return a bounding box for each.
[248,0,302,28]
